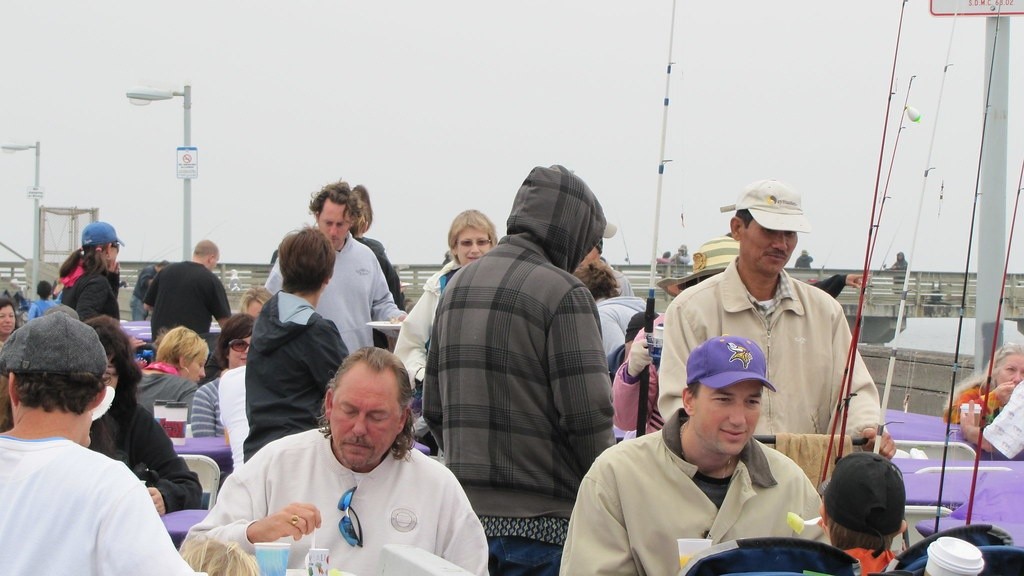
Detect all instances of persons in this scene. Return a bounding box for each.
[1,310,197,576]
[180,346,490,576]
[557,330,835,575]
[0,184,1024,514]
[425,163,618,576]
[800,449,915,575]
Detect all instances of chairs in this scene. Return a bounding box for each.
[171,452,220,511]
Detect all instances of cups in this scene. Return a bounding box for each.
[165,402,188,445]
[960,403,981,429]
[153,399,173,431]
[309,548,329,576]
[924,536,984,576]
[254,542,292,576]
[676,538,711,570]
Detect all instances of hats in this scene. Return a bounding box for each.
[686,333,776,393]
[1,312,109,386]
[602,222,617,239]
[82,222,125,248]
[817,452,905,535]
[719,178,811,235]
[656,238,742,294]
[624,312,661,342]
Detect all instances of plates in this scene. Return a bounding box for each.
[367,320,403,331]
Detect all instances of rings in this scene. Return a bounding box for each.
[291,515,300,527]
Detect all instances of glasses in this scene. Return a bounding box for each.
[228,339,253,353]
[458,238,490,247]
[337,486,363,549]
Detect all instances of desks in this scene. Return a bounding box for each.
[171,435,233,475]
[118,319,154,347]
[881,407,1023,551]
[160,509,212,552]
